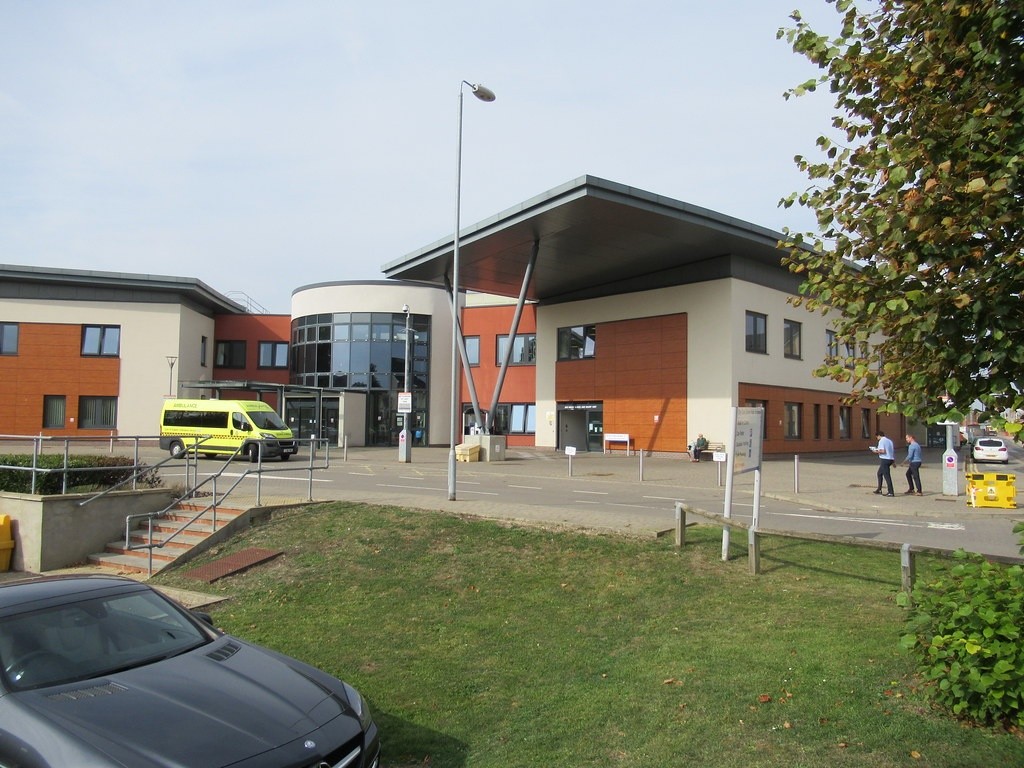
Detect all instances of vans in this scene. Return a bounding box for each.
[158,397,300,463]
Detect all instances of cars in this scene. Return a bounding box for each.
[970,438,1008,464]
[0,568,383,768]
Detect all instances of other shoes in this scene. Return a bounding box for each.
[904,489,915,495]
[916,492,923,496]
[882,492,895,497]
[873,489,882,494]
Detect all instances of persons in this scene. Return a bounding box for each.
[900,433,922,496]
[960,432,963,447]
[692,434,707,462]
[872,430,896,497]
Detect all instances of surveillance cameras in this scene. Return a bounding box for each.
[402,306,409,312]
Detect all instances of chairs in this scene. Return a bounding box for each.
[40,609,105,663]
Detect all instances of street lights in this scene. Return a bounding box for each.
[447,78,497,502]
[395,304,412,463]
[165,356,178,397]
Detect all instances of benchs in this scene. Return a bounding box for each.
[687,442,723,460]
